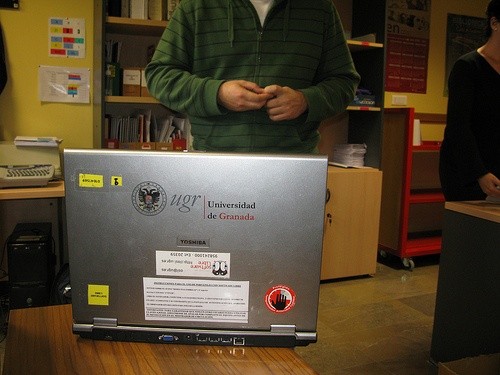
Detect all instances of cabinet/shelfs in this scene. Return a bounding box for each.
[102,0,387,282]
[378,106,446,258]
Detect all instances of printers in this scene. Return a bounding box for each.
[0,140,62,187]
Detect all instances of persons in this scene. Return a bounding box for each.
[145,0,360,156]
[438,0,500,202]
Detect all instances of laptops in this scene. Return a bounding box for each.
[63,149,329,348]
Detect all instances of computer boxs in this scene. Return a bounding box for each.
[6,221,58,311]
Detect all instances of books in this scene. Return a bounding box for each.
[106,40,121,63]
[334,144,366,166]
[105,114,186,143]
[14,136,58,146]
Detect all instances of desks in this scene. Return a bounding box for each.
[0,180,65,328]
[430,201,500,367]
[2,303,317,375]
